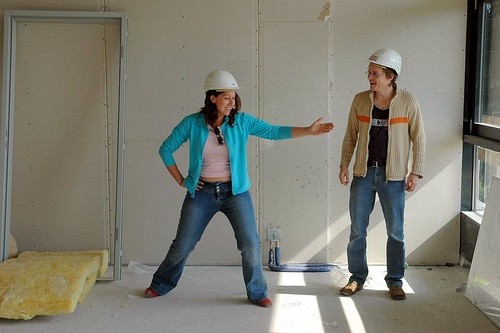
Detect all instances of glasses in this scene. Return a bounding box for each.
[365,72,391,78]
[214,127,223,145]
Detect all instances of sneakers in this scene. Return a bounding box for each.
[340,281,362,296]
[389,285,406,300]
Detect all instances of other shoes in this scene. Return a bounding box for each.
[258,298,272,307]
[144,287,158,298]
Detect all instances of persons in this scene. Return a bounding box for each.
[338,48,427,300]
[144,70,336,308]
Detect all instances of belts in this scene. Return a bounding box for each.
[367,161,386,167]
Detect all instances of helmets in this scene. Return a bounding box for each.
[204,70,241,93]
[368,48,401,75]
